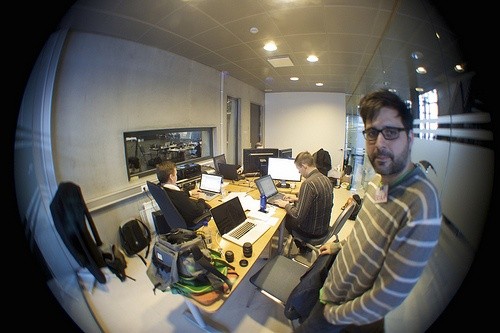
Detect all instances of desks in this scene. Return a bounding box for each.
[178,172,304,333]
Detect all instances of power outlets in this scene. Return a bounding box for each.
[141,184,149,191]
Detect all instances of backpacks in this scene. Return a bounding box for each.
[120,219,151,257]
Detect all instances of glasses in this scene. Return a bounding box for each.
[361,127,407,141]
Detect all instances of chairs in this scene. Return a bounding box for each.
[146,181,212,231]
[246,197,359,333]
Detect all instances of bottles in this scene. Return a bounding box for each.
[202,221,212,249]
[261,194,266,210]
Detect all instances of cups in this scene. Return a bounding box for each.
[225,251,235,263]
[243,242,252,258]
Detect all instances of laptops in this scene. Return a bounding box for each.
[193,173,222,202]
[254,175,291,206]
[209,196,271,246]
[218,163,245,181]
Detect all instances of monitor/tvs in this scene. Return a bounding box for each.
[214,155,226,174]
[243,149,302,187]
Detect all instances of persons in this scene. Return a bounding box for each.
[272,151,334,252]
[155,160,205,226]
[291,88,443,333]
